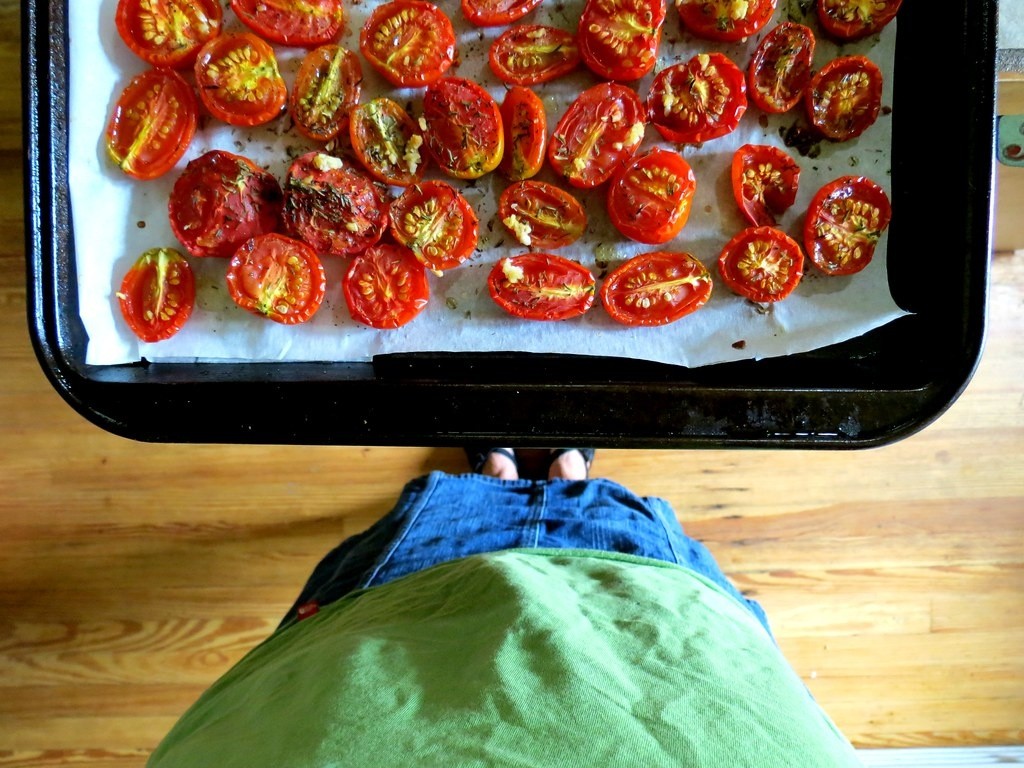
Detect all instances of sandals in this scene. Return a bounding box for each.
[542,449,594,480]
[465,447,518,474]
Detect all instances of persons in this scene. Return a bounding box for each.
[143,444,854,768]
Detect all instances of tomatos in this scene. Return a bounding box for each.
[104,0,890,343]
